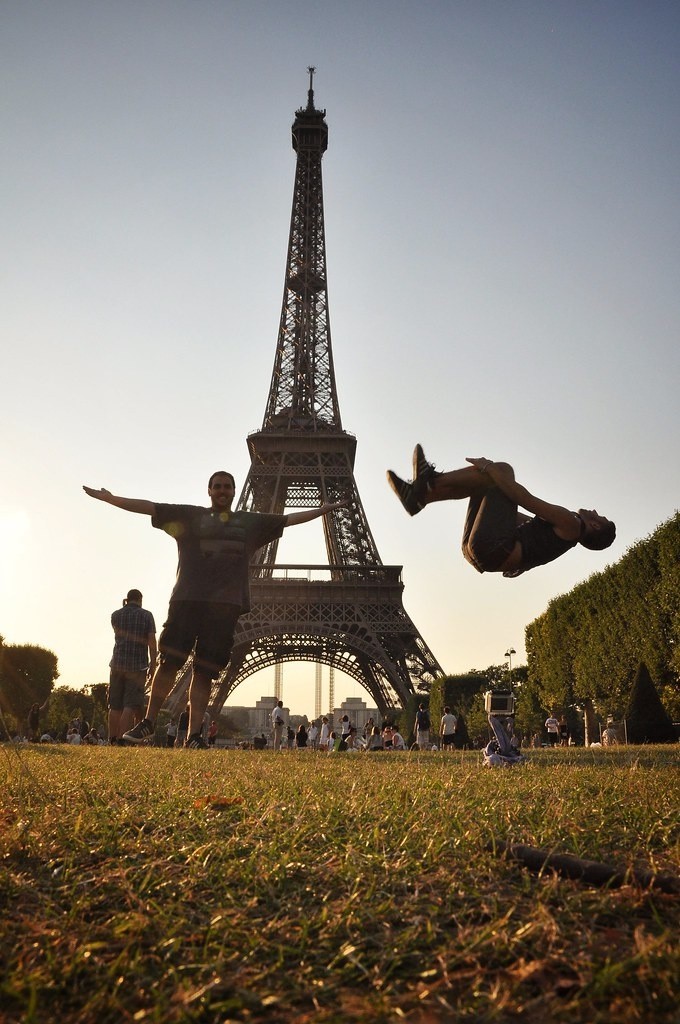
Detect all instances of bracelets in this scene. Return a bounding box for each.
[480,460,493,473]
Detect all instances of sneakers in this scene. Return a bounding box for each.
[184,734,210,749]
[388,471,426,516]
[414,444,443,490]
[123,716,155,742]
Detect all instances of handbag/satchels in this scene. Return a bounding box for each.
[338,740,348,751]
[362,731,366,739]
[384,740,393,747]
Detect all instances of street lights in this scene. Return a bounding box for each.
[504,646,516,694]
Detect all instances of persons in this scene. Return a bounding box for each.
[385,443,616,580]
[270,700,616,767]
[106,588,159,748]
[82,471,349,750]
[162,715,267,750]
[5,701,105,747]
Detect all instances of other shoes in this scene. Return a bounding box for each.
[108,736,118,746]
[118,739,135,746]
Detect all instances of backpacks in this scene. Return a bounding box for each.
[418,711,430,730]
